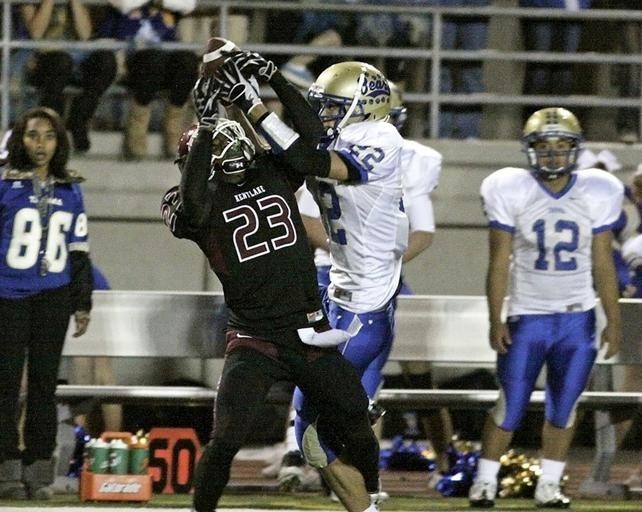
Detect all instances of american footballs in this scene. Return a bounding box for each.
[203,37,260,112]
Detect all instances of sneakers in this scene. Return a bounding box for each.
[468,479,497,507]
[533,481,570,508]
[24,482,54,500]
[0,481,27,500]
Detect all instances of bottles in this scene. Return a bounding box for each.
[82,436,151,477]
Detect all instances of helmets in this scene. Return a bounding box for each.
[178,119,255,186]
[386,79,408,131]
[309,61,389,137]
[521,107,583,181]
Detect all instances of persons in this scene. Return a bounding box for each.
[262,79,460,499]
[0,105,94,498]
[1,1,642,160]
[575,148,642,502]
[69,263,126,437]
[158,47,381,511]
[213,56,407,512]
[467,107,627,510]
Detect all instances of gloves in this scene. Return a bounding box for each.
[230,50,279,83]
[214,59,262,116]
[190,77,221,128]
[72,309,89,338]
